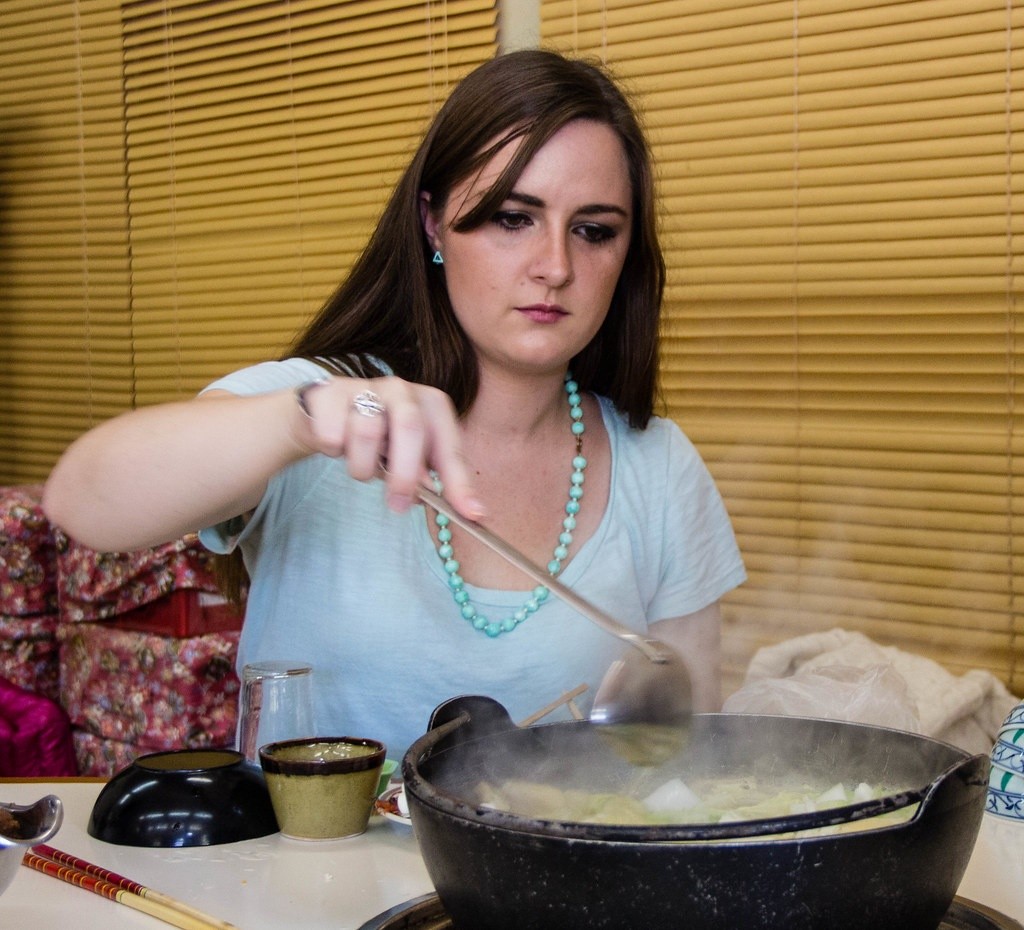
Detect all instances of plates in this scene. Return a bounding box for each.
[374,786,412,827]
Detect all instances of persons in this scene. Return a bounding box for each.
[43,48,746,770]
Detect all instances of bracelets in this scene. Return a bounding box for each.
[293,374,331,396]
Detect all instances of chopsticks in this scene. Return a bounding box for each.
[22,841,244,930]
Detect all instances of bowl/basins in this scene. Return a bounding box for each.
[87,746,278,848]
[257,735,387,841]
[373,758,398,796]
[984,698,1024,822]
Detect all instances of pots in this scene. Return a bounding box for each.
[398,689,995,930]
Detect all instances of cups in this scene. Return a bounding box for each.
[233,659,320,768]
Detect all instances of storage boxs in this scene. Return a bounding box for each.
[2,480,249,778]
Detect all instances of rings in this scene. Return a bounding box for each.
[354,389,387,416]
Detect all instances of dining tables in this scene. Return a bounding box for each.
[0,774,1023,930]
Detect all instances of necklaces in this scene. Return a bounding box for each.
[429,374,587,635]
